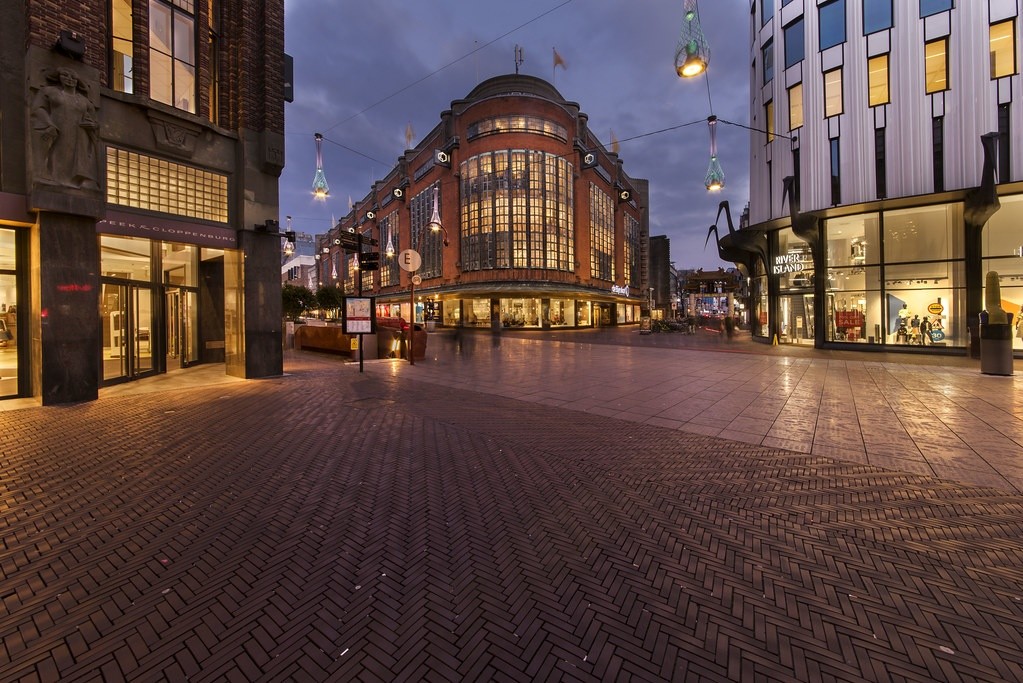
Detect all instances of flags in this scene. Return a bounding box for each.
[612,134,621,154]
[555,50,566,68]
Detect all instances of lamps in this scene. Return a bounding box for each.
[674,0,711,78]
[311,133,330,196]
[283,216,395,285]
[705,114,726,189]
[430,186,441,230]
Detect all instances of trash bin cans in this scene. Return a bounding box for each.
[978,323,1015,375]
[426,320,437,332]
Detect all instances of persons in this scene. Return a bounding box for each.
[897,303,934,345]
[31,66,100,191]
[1016,304,1023,341]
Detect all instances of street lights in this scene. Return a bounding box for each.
[409,221,451,365]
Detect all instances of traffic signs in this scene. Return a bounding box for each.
[334,228,380,272]
